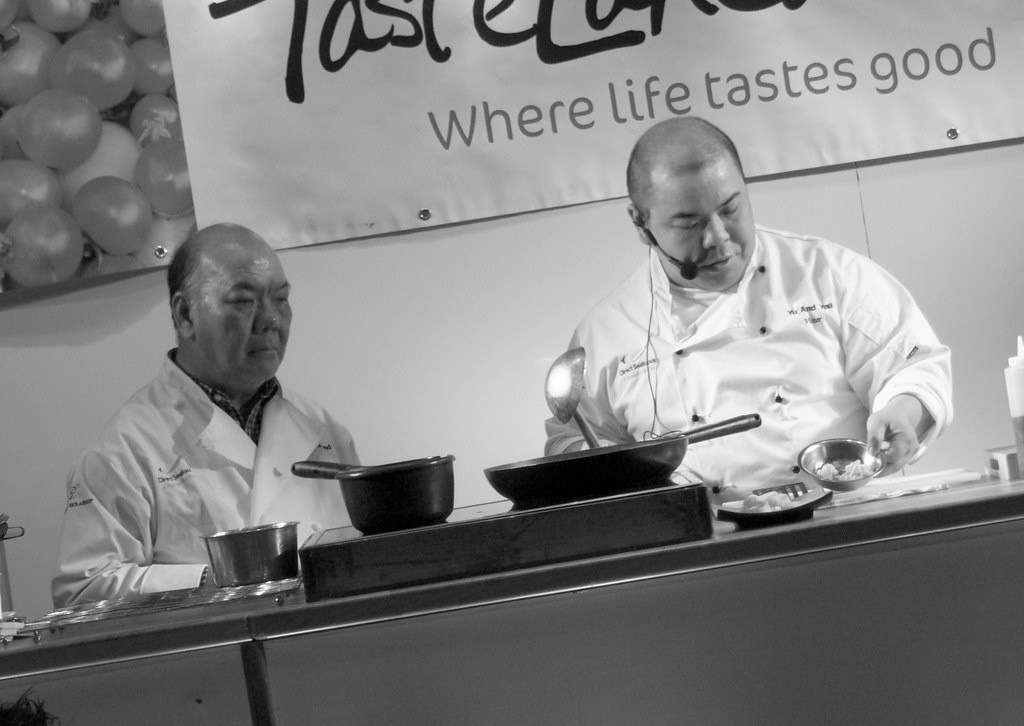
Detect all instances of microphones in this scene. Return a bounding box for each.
[644,227,698,280]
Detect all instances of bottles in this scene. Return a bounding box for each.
[1004,336,1024,480]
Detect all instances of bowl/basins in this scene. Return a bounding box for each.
[797,438,886,491]
[200,520,301,588]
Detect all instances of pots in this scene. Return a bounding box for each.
[481,413,763,507]
[289,454,456,535]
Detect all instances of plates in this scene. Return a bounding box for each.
[717,488,832,530]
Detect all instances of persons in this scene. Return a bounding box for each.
[545,116,954,506]
[51,223,361,609]
